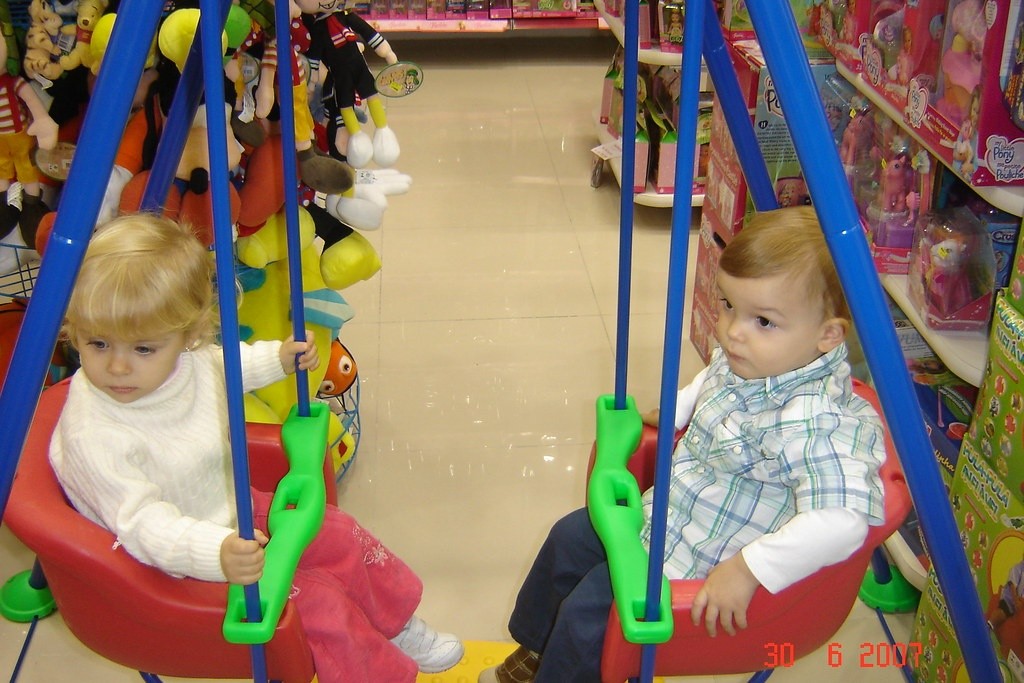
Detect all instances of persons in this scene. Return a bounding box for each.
[48,213,464,683]
[478,208,888,683]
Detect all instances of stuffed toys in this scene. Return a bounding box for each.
[0,0,423,474]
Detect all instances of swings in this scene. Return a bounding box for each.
[3,1,339,683]
[586,0,915,683]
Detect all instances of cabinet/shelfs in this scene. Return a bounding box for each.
[831,59,1023,591]
[596,0,709,207]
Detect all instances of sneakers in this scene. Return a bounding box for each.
[477,646,542,683]
[388,615,464,674]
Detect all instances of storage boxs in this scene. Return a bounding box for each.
[690,0,856,366]
[600,43,624,125]
[702,38,854,247]
[604,54,652,193]
[720,1,757,43]
[619,0,652,49]
[642,94,678,194]
[903,217,1024,683]
[689,210,728,366]
